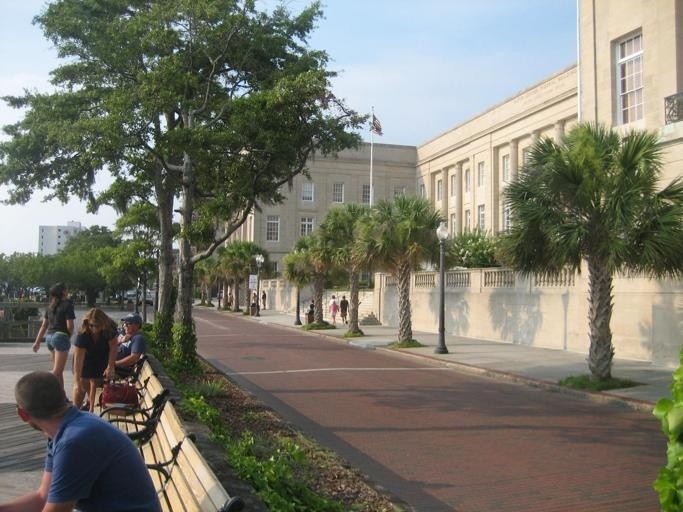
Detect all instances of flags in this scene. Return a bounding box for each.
[370,115,386,136]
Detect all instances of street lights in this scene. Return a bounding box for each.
[433,221,449,354]
[255,254,264,317]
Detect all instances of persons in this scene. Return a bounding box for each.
[329,301,339,326]
[79,312,144,411]
[340,296,350,324]
[0,370,164,512]
[71,307,119,414]
[227,290,266,309]
[308,300,314,310]
[33,281,76,403]
[330,295,339,305]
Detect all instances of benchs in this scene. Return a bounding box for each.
[99,352,245,512]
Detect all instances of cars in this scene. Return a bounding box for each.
[115,287,154,306]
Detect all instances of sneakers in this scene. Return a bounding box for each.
[80,399,89,411]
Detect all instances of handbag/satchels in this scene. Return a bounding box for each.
[101,384,138,415]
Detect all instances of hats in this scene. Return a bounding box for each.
[121,313,141,324]
